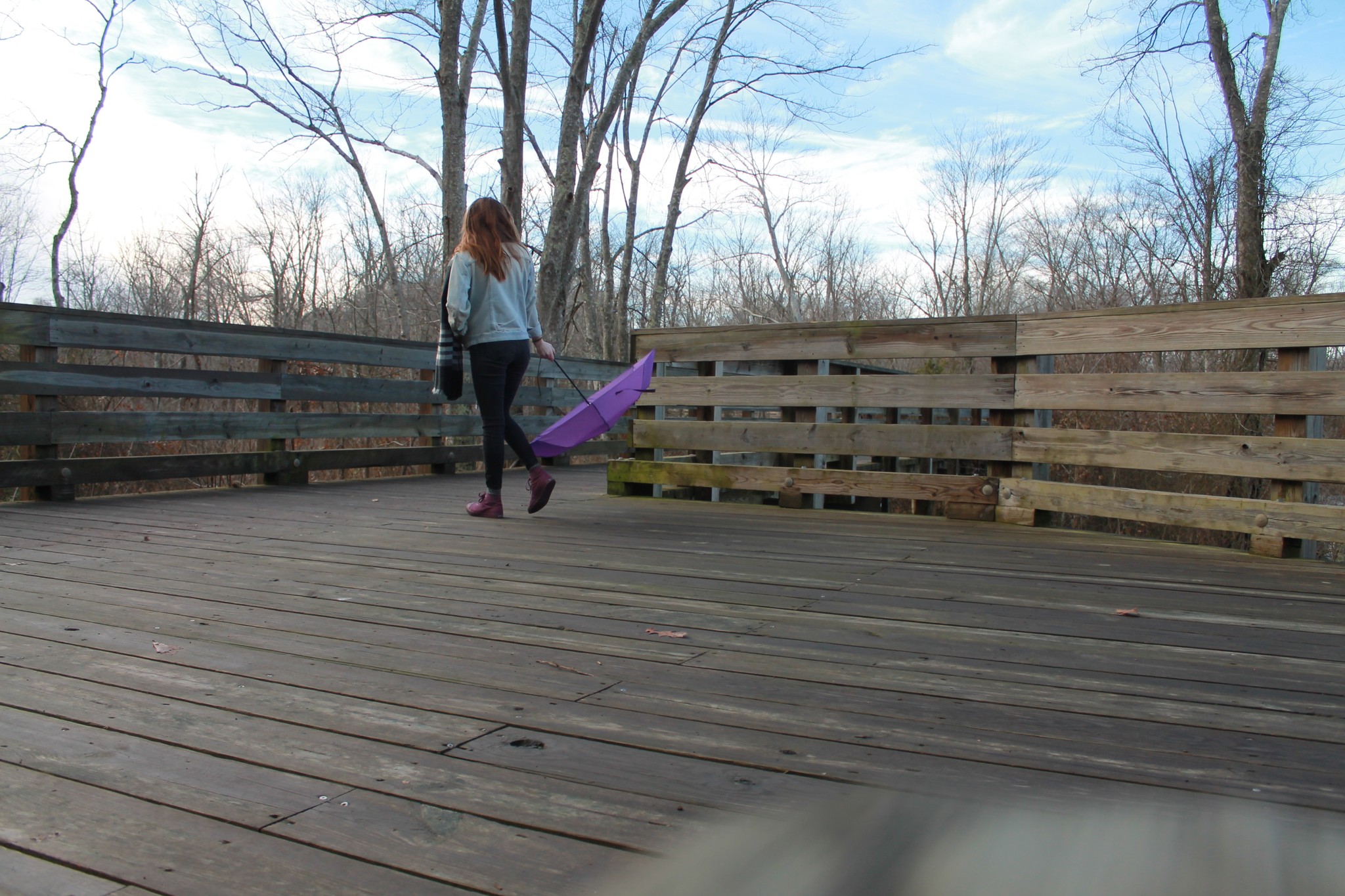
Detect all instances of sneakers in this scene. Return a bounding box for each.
[526,465,556,514]
[466,490,504,519]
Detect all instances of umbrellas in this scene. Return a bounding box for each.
[530,350,657,455]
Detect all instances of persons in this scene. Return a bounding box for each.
[432,197,556,517]
